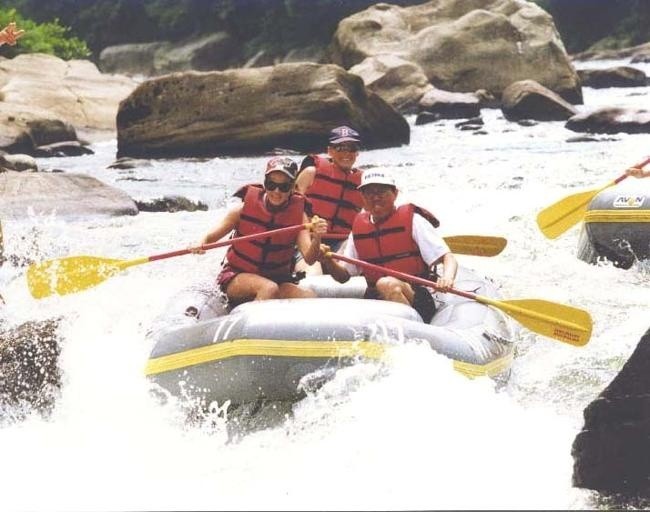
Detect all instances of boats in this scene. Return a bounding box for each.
[147,258,523,420]
[585,177,650,272]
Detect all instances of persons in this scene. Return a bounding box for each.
[0,21,26,47]
[187,154,329,301]
[318,166,458,324]
[295,125,369,254]
[625,167,650,179]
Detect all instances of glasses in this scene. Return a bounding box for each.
[264,178,294,192]
[330,142,359,152]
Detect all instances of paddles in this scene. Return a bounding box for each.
[28,217,322,300]
[320,246,592,347]
[323,232,506,257]
[537,159,649,240]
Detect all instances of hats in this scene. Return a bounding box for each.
[355,167,397,189]
[265,157,298,180]
[328,125,361,146]
